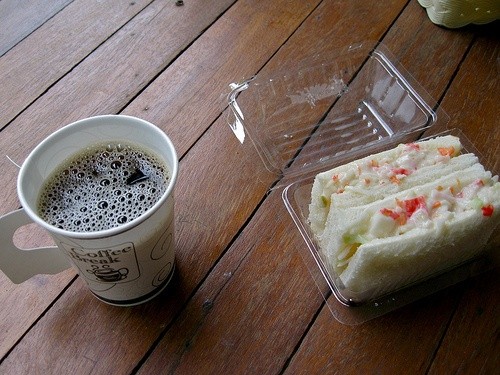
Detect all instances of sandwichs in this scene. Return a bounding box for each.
[308,134,500,299]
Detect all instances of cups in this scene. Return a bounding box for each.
[16,111,177,310]
